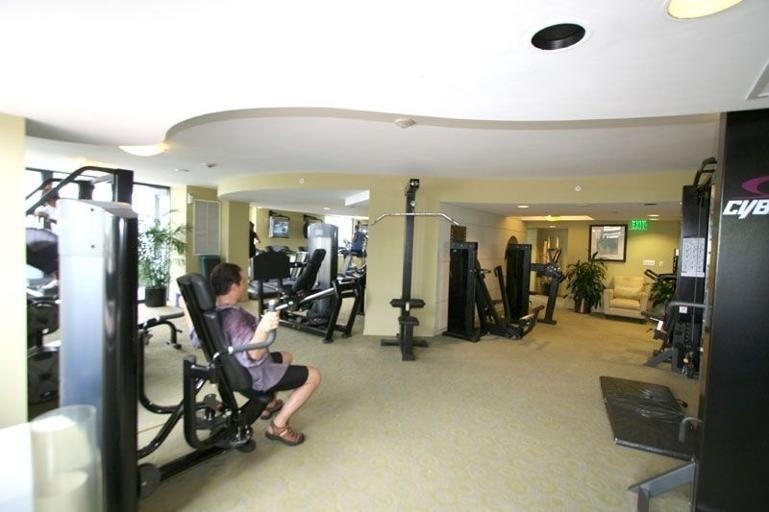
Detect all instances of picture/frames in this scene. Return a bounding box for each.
[589,224,627,262]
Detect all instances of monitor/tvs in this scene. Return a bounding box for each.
[269,216,290,238]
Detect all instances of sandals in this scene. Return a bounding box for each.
[265,420,303,445]
[261,399,283,419]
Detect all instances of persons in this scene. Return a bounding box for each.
[247,221,262,279]
[349,224,367,268]
[188,261,323,447]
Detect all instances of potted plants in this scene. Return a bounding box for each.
[138,209,193,308]
[562,251,609,314]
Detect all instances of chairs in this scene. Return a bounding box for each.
[603,276,649,324]
